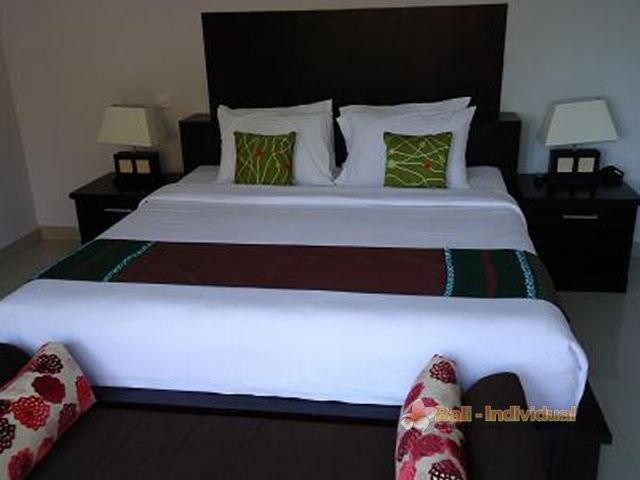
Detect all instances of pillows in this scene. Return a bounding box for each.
[394,353,466,480]
[0,330,99,480]
[214,96,479,192]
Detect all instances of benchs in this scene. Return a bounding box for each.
[0,343,540,480]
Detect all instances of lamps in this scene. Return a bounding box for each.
[534,97,621,186]
[97,104,170,190]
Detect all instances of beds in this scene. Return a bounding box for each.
[0,111,589,421]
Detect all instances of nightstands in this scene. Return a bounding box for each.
[519,173,640,295]
[67,170,183,245]
